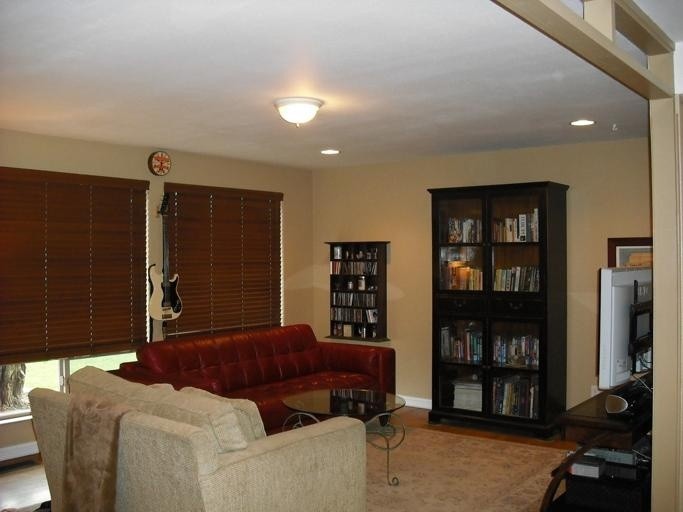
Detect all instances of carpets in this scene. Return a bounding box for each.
[364,422,568,511]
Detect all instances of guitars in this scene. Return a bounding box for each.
[149,192,185,322]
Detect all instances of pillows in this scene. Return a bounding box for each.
[180,387,267,442]
[146,383,174,391]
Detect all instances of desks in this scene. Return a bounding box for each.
[539,372,653,512]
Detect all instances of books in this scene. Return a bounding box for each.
[330,390,381,418]
[332,261,377,340]
[439,208,540,421]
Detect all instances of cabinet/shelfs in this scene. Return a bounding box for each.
[323,240,390,343]
[330,389,385,417]
[427,180,568,440]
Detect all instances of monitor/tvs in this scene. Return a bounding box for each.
[596,266,652,391]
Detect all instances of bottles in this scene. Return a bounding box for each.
[357,276,365,292]
[347,281,354,290]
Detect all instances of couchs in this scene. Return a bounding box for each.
[28,365,368,512]
[117,323,396,436]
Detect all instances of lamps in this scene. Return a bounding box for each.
[271,98,324,128]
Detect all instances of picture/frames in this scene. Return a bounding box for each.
[607,236,653,269]
